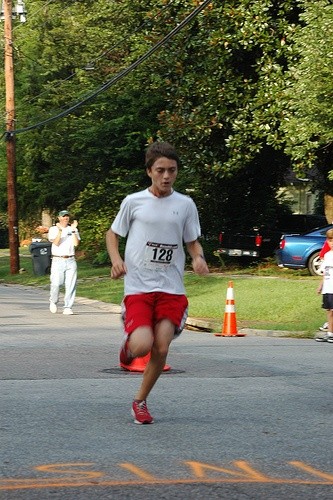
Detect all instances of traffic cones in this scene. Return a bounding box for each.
[214,283,246,337]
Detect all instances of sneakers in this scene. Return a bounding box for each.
[119,336,135,365]
[128,398,155,424]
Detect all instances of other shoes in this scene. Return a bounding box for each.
[320,322,328,331]
[49,303,57,313]
[63,308,74,315]
[315,332,333,342]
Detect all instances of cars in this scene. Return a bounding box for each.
[274,223,333,277]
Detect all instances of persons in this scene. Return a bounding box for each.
[315,228,333,343]
[106,142,209,424]
[48,210,81,315]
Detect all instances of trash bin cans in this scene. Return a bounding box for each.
[30,241,52,276]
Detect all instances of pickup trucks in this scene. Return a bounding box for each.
[217,214,329,267]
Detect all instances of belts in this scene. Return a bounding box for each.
[52,255,75,258]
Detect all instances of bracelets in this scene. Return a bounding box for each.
[72,231,75,233]
[200,255,205,259]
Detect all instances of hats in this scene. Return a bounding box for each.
[59,210,69,217]
